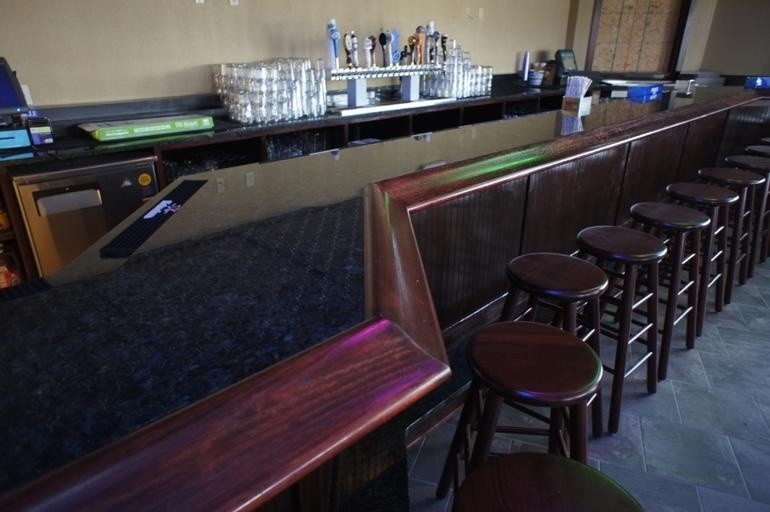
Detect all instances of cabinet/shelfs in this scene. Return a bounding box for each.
[0,65,737,282]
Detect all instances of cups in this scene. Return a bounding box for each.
[210,56,327,124]
[421,40,493,98]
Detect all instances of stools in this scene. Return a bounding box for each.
[725,154,770,264]
[438,321,605,512]
[549,226,667,433]
[599,201,712,378]
[665,183,740,338]
[699,166,766,305]
[744,143,770,159]
[761,134,769,145]
[454,450,644,512]
[480,251,610,449]
